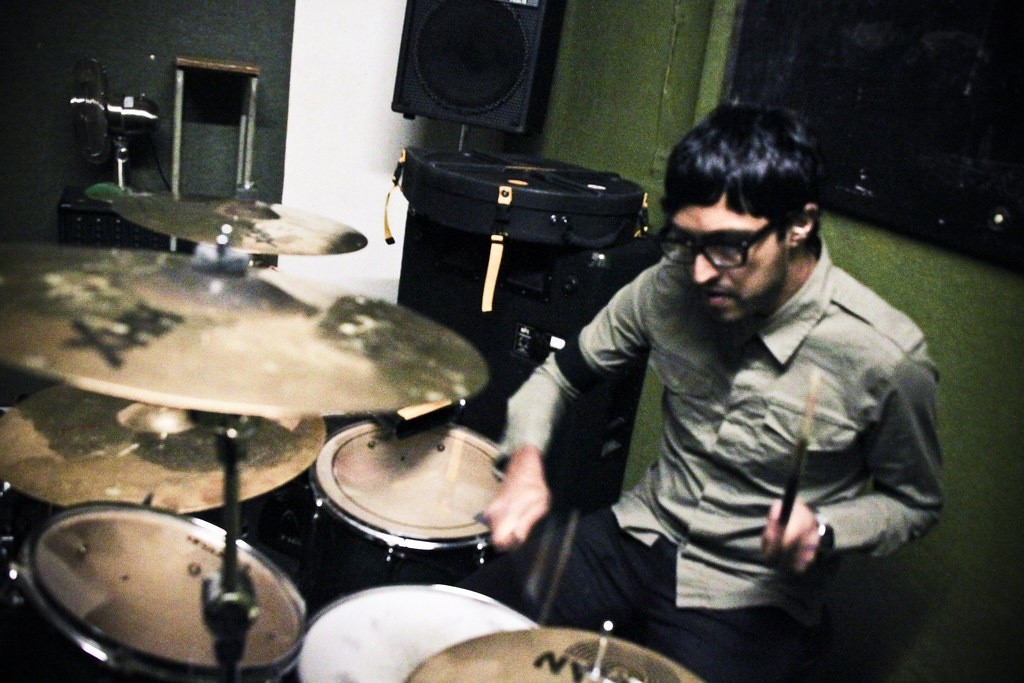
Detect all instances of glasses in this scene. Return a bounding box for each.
[655,219,784,270]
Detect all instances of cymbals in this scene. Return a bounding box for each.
[110,192,370,256]
[401,627,706,683]
[1,384,328,515]
[0,247,491,418]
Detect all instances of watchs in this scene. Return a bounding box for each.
[816,521,833,558]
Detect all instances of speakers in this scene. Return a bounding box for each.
[392,0,568,136]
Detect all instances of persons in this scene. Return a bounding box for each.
[456,103,943,683]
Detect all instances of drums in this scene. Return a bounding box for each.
[309,415,509,581]
[293,583,543,683]
[15,499,305,683]
